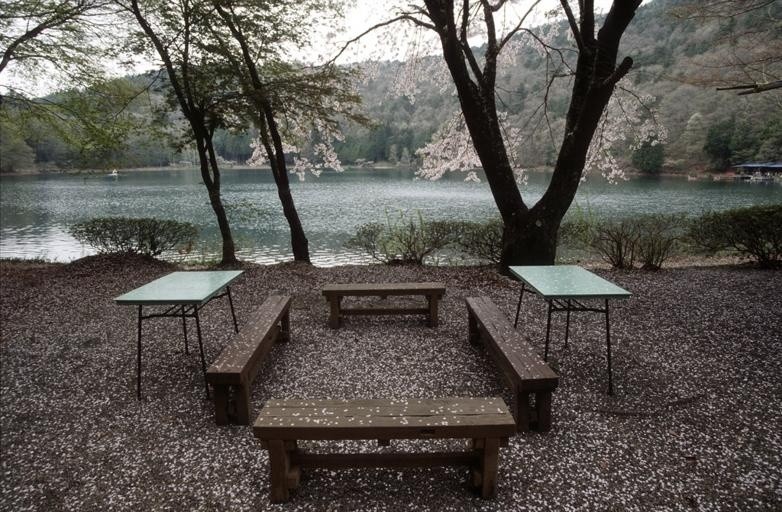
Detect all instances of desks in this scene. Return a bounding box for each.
[112,271,244,400]
[508,264,634,396]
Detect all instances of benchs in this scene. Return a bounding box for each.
[253,398,516,503]
[204,294,292,426]
[463,296,559,431]
[322,282,446,328]
